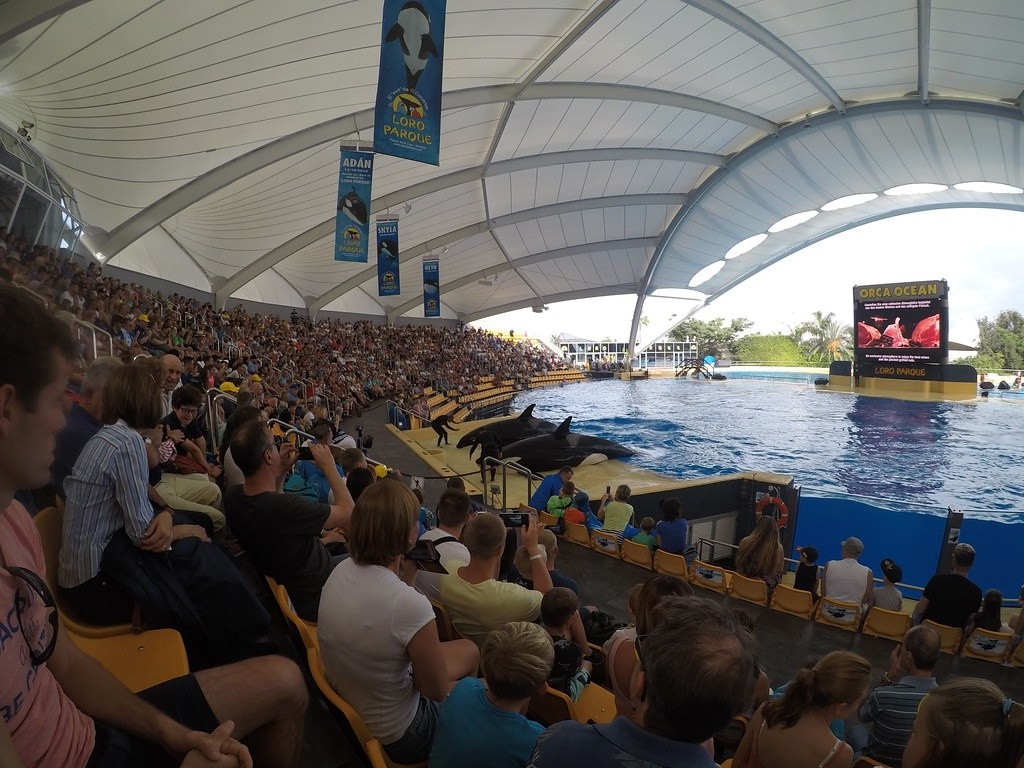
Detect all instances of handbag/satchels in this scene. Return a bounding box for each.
[581,610,615,646]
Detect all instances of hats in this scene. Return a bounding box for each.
[841,537,863,555]
[795,545,818,563]
[220,381,241,393]
[881,559,902,583]
[251,374,262,382]
[290,384,299,389]
[139,315,151,323]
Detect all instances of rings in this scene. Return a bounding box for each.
[162,545,167,549]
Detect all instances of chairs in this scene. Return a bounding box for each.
[424,376,524,424]
[66,627,189,695]
[271,423,297,475]
[528,370,589,389]
[265,504,1024,768]
[34,507,139,638]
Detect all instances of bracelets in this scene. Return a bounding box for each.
[574,640,587,647]
[529,554,542,560]
[882,672,894,685]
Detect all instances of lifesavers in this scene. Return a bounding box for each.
[755,496,789,528]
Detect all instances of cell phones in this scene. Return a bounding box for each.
[498,513,529,528]
[403,540,439,560]
[607,486,610,495]
[298,447,315,461]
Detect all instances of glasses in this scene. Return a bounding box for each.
[262,435,282,464]
[4,566,59,665]
[180,407,199,416]
[633,634,650,703]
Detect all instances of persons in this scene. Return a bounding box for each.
[0,228,1024,768]
